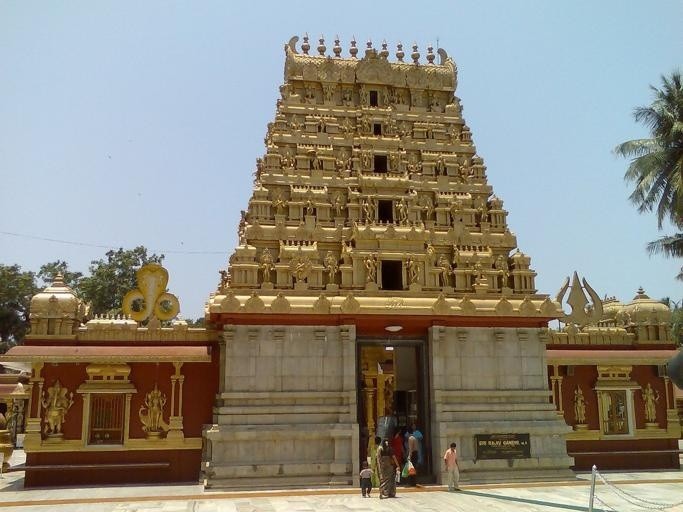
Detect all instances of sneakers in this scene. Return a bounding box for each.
[447,487,462,492]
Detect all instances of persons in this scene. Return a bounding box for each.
[359,422,424,500]
[218,84,509,289]
[443,442,462,492]
